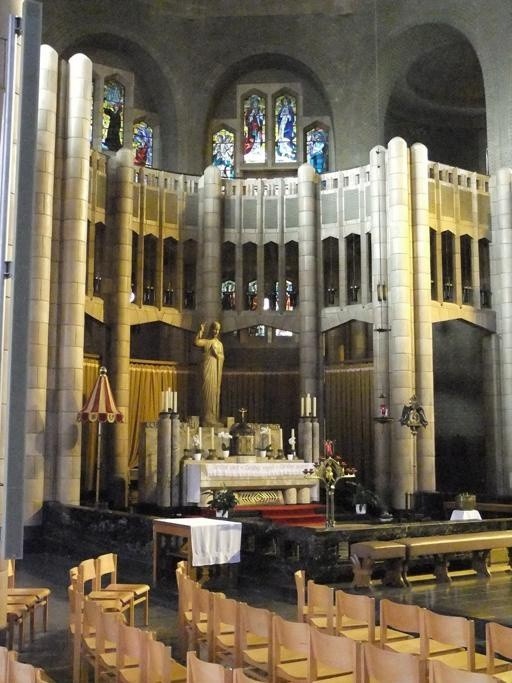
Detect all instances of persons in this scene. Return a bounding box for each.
[308,126,327,174]
[194,320,226,428]
[104,103,123,151]
[212,134,234,178]
[276,96,296,162]
[242,95,265,165]
[133,121,153,168]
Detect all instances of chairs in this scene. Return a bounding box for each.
[2,553,511,683]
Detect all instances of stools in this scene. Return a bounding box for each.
[350,540,405,590]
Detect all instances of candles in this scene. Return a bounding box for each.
[291,428,295,449]
[299,391,317,419]
[198,426,202,450]
[185,425,190,449]
[211,426,214,449]
[278,427,282,449]
[268,429,272,449]
[158,383,178,414]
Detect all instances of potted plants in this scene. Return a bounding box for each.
[198,478,240,520]
[346,479,382,517]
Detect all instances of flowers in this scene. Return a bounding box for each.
[217,430,232,450]
[191,433,201,453]
[253,426,273,453]
[287,435,297,445]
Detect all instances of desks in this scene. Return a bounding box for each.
[152,517,242,588]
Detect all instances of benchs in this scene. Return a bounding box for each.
[397,530,512,588]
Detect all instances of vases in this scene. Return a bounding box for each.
[286,453,294,460]
[193,452,202,460]
[221,449,230,458]
[259,449,267,458]
